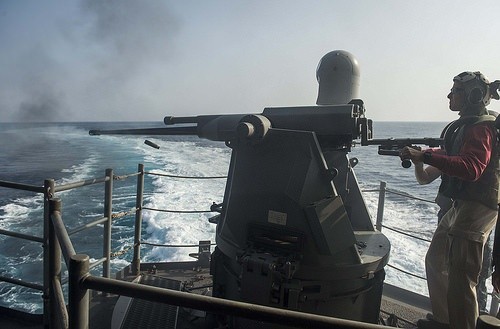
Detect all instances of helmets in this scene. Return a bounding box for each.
[453,70,491,114]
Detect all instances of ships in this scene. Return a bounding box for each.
[0,50,500,329]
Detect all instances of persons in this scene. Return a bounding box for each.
[480,210,500,316]
[397,70,500,329]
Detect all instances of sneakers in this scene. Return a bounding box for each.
[417,313,454,328]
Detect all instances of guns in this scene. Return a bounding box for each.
[88,104,444,170]
